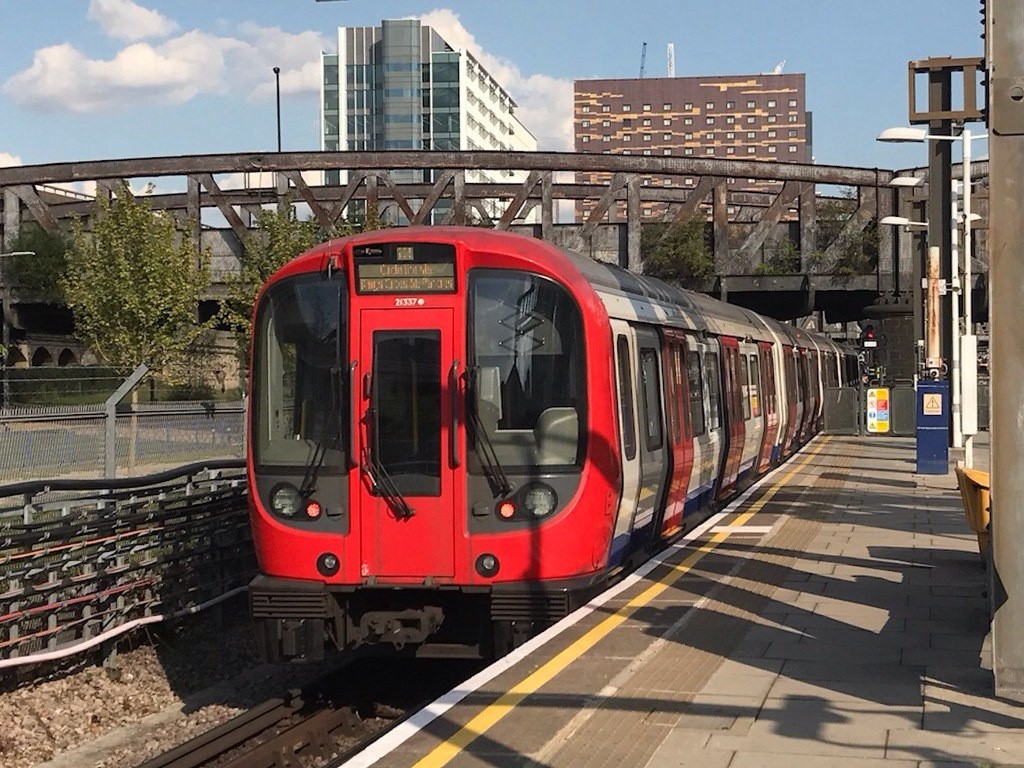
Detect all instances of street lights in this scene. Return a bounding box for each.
[876,126,988,484]
[273,65,282,153]
[881,173,982,451]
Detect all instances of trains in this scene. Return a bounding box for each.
[241,220,870,678]
